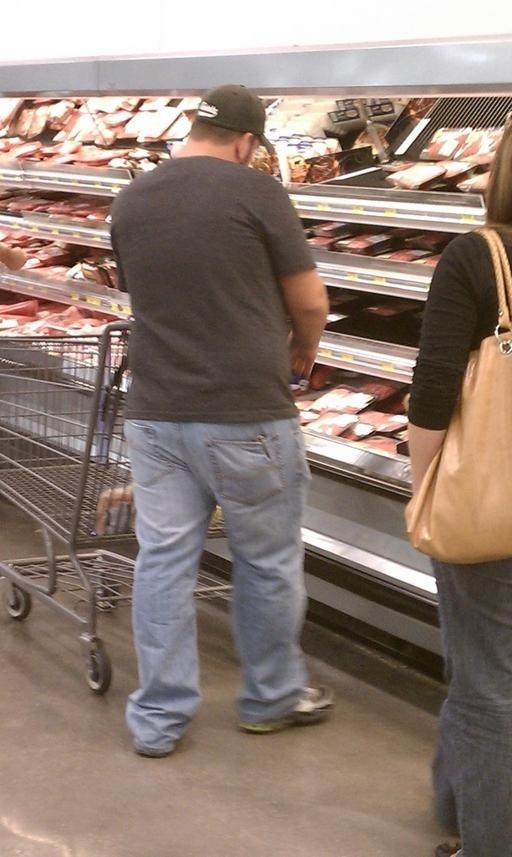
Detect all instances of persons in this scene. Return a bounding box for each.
[0,242,27,271]
[408,115,512,857]
[111,84,334,758]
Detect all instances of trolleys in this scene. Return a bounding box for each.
[0,316,236,697]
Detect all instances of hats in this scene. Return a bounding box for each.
[195,84,275,154]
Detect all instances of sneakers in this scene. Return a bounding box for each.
[239,684,336,732]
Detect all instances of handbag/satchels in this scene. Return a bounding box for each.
[402,329,512,565]
